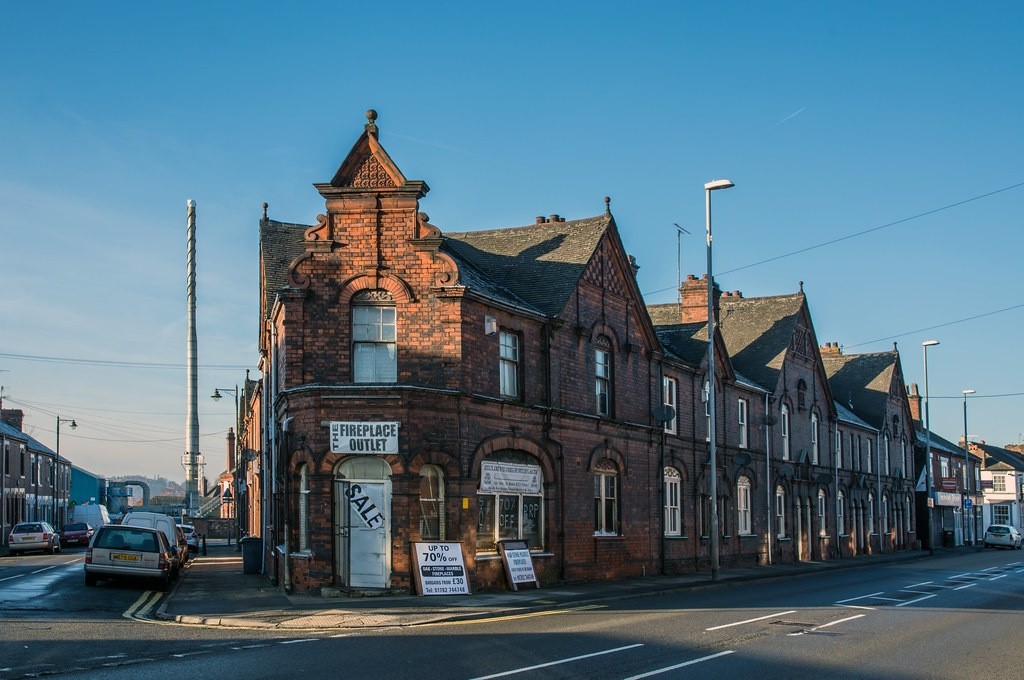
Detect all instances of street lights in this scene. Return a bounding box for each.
[211,385,244,550]
[963,389,977,551]
[54,415,77,532]
[703,183,736,581]
[922,339,940,558]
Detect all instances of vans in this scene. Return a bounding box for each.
[121,512,190,580]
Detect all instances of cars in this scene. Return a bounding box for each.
[984,524,1022,550]
[61,522,99,548]
[8,521,61,555]
[83,524,180,589]
[176,524,202,552]
